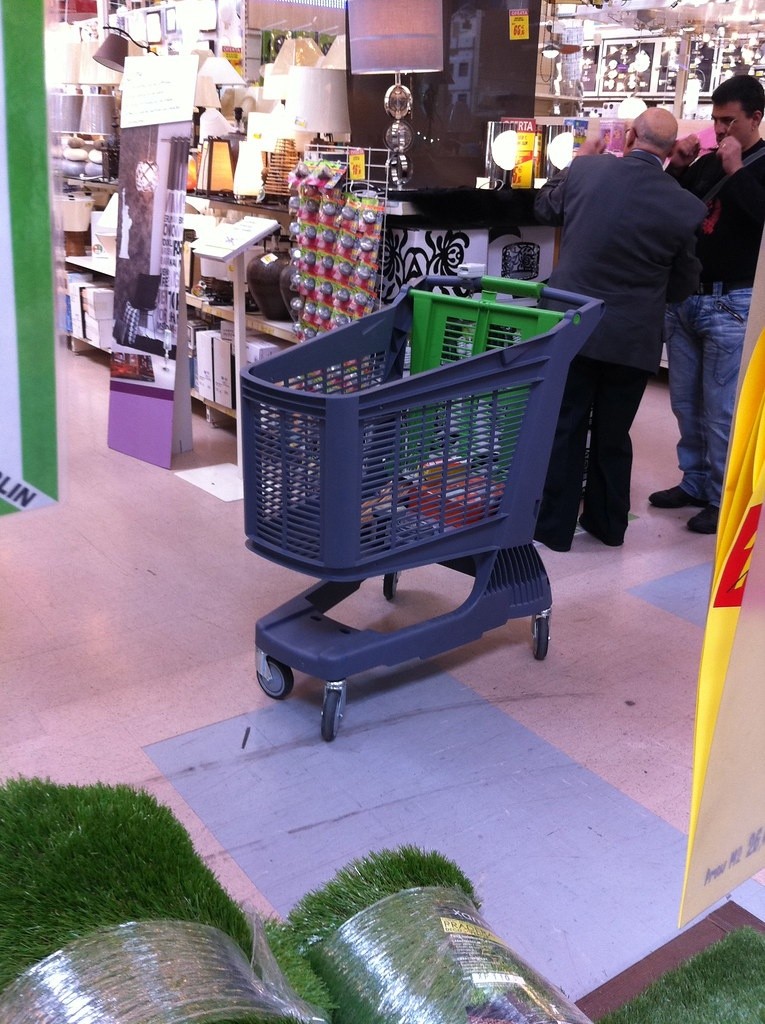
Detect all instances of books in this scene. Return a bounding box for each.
[194,216,278,263]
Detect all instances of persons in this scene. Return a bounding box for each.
[537,107,710,554]
[648,73,765,533]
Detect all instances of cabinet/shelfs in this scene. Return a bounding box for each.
[54,179,412,474]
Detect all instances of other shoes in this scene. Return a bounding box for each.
[579,513,624,546]
[534,534,571,551]
[648,486,710,508]
[688,504,719,533]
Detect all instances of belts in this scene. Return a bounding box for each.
[696,278,754,295]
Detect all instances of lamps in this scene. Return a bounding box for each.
[44,0,447,203]
[473,120,528,190]
[542,130,578,173]
[536,0,765,105]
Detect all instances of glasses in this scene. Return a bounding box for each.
[696,119,738,151]
[625,128,638,139]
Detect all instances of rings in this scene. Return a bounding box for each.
[721,144,726,149]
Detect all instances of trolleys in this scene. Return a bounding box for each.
[238,273,607,742]
